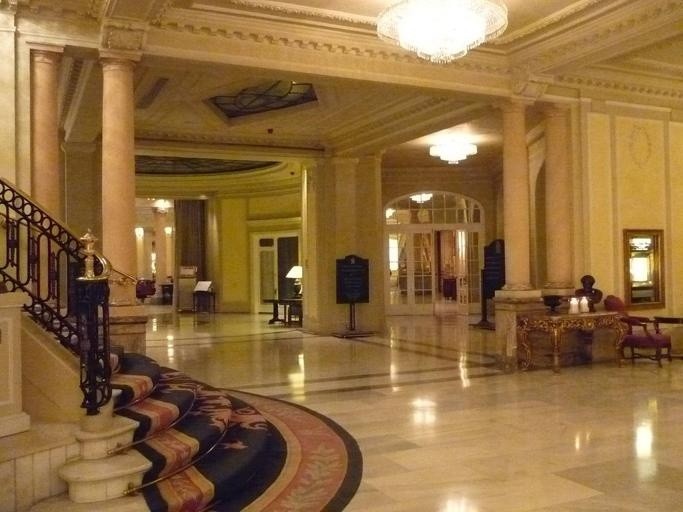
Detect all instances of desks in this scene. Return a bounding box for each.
[652,310,682,360]
[263,297,303,325]
[518,308,630,373]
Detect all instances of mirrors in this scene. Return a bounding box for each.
[625,227,666,312]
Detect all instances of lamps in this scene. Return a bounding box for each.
[147,198,173,214]
[410,191,435,206]
[374,0,507,64]
[429,142,478,164]
[285,265,304,298]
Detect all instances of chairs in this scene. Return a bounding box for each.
[604,294,672,367]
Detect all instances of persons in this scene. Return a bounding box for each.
[575,274,603,305]
